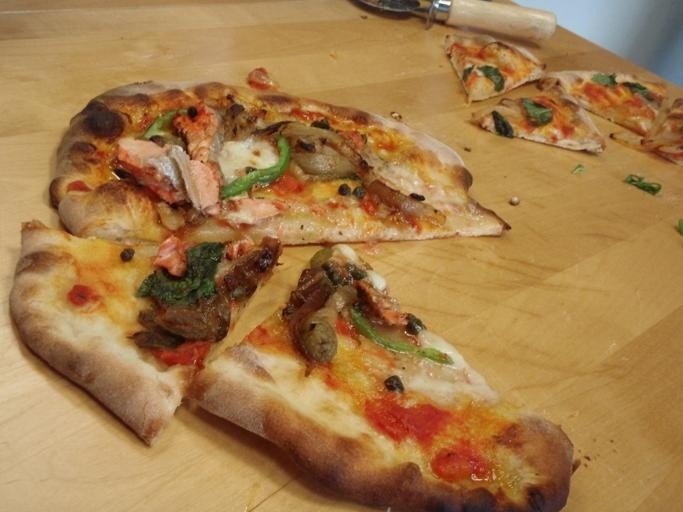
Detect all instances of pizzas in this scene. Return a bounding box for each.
[442,34,683,166]
[8,66,584,512]
